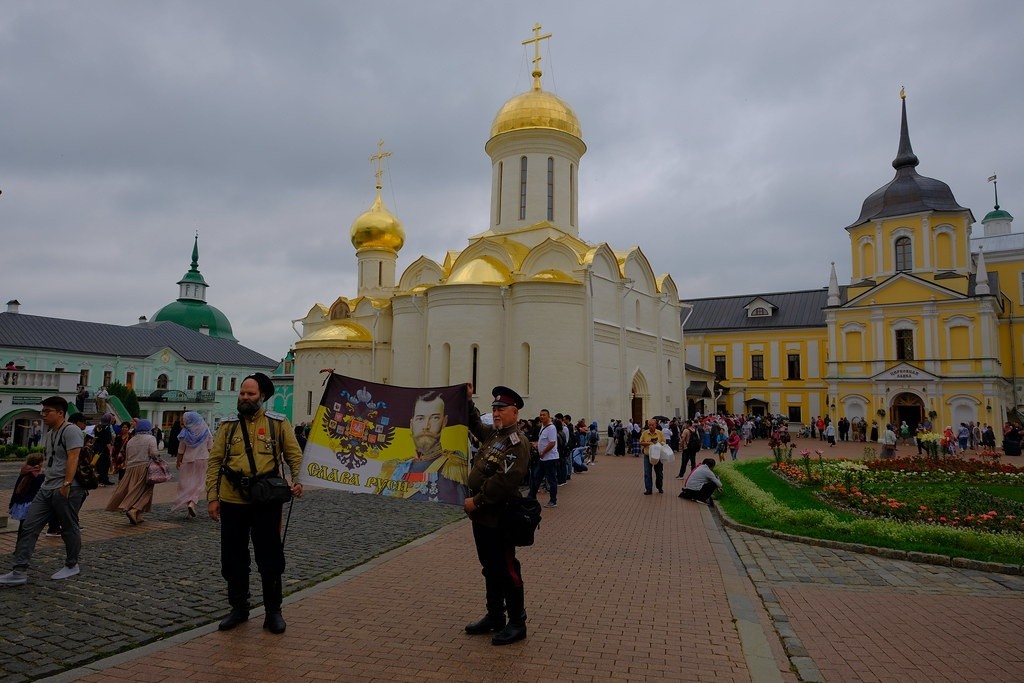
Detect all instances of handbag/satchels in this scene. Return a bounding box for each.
[485,495,541,548]
[886,444,894,450]
[148,456,172,483]
[116,453,125,465]
[239,477,291,508]
[648,442,676,466]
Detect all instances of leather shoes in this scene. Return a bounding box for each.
[465,614,506,634]
[218,606,249,631]
[492,620,527,645]
[264,605,286,634]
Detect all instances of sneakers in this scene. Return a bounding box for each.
[79,525,85,530]
[46,527,62,537]
[0,568,27,584]
[51,564,80,579]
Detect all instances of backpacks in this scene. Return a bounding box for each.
[58,424,94,480]
[558,432,570,457]
[590,431,597,445]
[685,428,701,452]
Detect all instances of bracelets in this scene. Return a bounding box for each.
[63,482,72,487]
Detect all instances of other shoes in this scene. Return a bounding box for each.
[527,473,573,507]
[98,480,115,487]
[643,474,711,506]
[126,510,145,524]
[186,504,197,520]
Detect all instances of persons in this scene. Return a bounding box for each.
[639,419,666,495]
[463,383,531,645]
[3,360,17,384]
[675,421,699,480]
[74,386,89,412]
[8,452,46,553]
[20,420,42,449]
[605,411,789,461]
[371,391,470,507]
[0,396,87,584]
[518,409,599,508]
[880,417,994,458]
[1002,421,1024,449]
[96,386,109,414]
[295,425,307,452]
[811,414,868,446]
[870,422,879,443]
[170,412,213,518]
[684,458,722,505]
[205,372,304,635]
[46,412,183,537]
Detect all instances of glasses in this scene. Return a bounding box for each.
[40,409,60,416]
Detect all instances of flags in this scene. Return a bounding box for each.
[298,374,472,507]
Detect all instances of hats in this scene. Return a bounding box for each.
[67,412,94,425]
[490,385,524,410]
